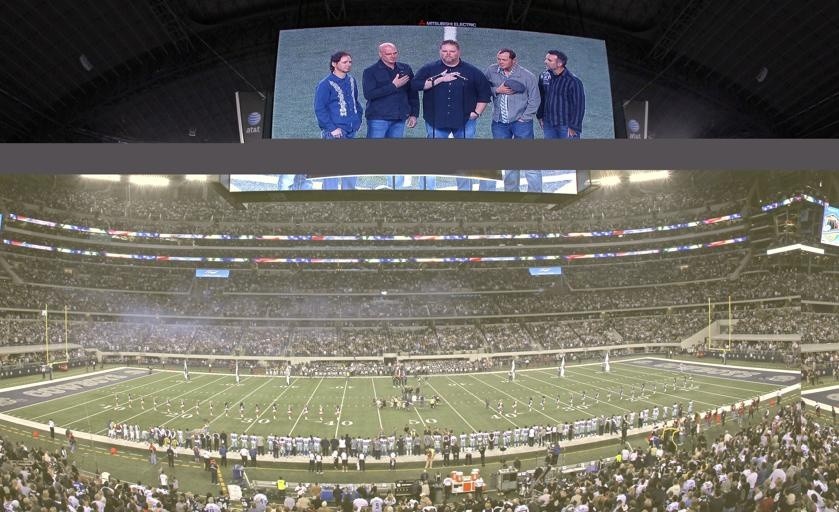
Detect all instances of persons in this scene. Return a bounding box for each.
[313,52,363,138]
[484,48,542,139]
[408,40,493,140]
[358,42,421,138]
[535,49,586,139]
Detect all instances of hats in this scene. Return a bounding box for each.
[504,79,526,95]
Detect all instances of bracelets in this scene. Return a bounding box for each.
[474,112,481,117]
